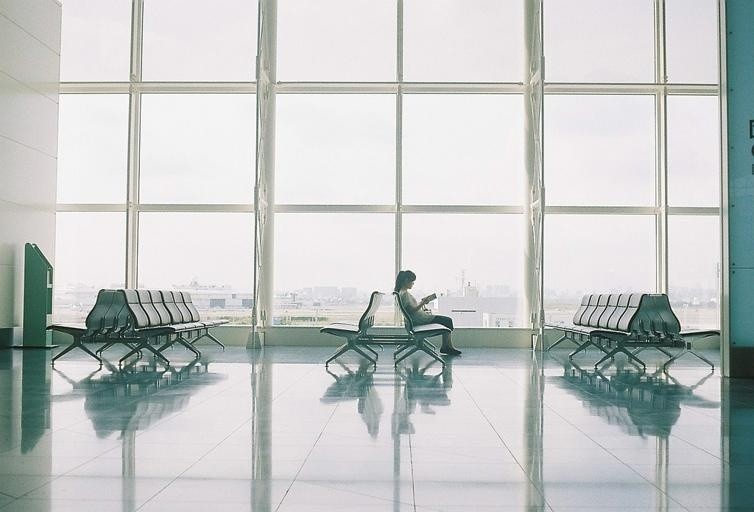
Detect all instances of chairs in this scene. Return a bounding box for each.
[319,290,452,372]
[46,287,230,366]
[52,364,226,441]
[542,352,720,440]
[542,292,714,373]
[321,359,451,437]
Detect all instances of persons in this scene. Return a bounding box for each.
[396,353,461,435]
[391,269,462,355]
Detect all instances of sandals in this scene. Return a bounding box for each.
[440,347,461,354]
